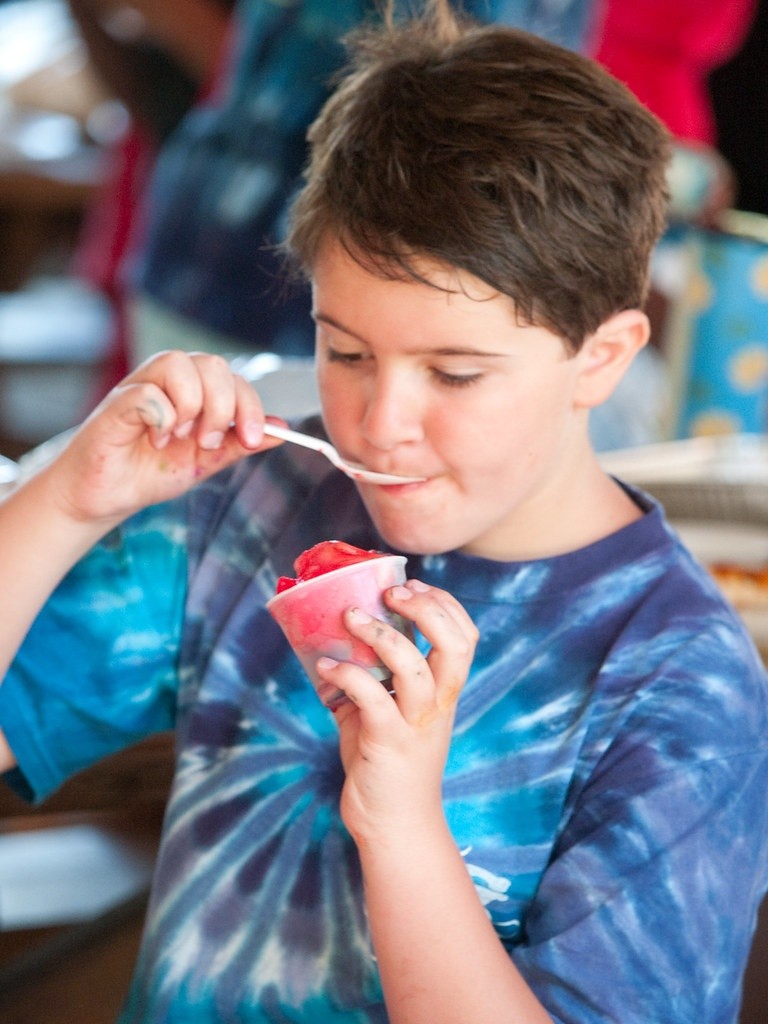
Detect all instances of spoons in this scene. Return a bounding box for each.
[227,419,426,486]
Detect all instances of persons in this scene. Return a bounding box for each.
[0,24,768,1024]
[0,0,756,392]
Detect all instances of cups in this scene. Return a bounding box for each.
[264,556,420,714]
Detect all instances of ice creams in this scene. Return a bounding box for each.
[266,540,416,712]
